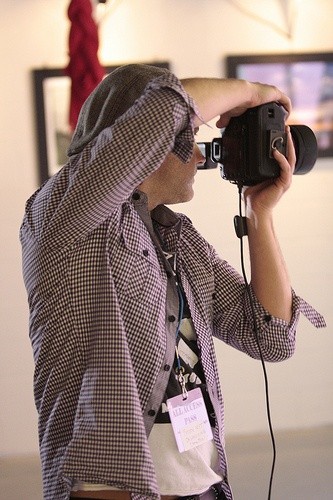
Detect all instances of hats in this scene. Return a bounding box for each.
[66,64,176,157]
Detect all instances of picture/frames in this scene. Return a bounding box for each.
[225,52,333,158]
[31,61,175,187]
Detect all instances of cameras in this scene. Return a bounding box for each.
[196,102,318,186]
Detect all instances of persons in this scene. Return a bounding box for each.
[19,64,327,500]
